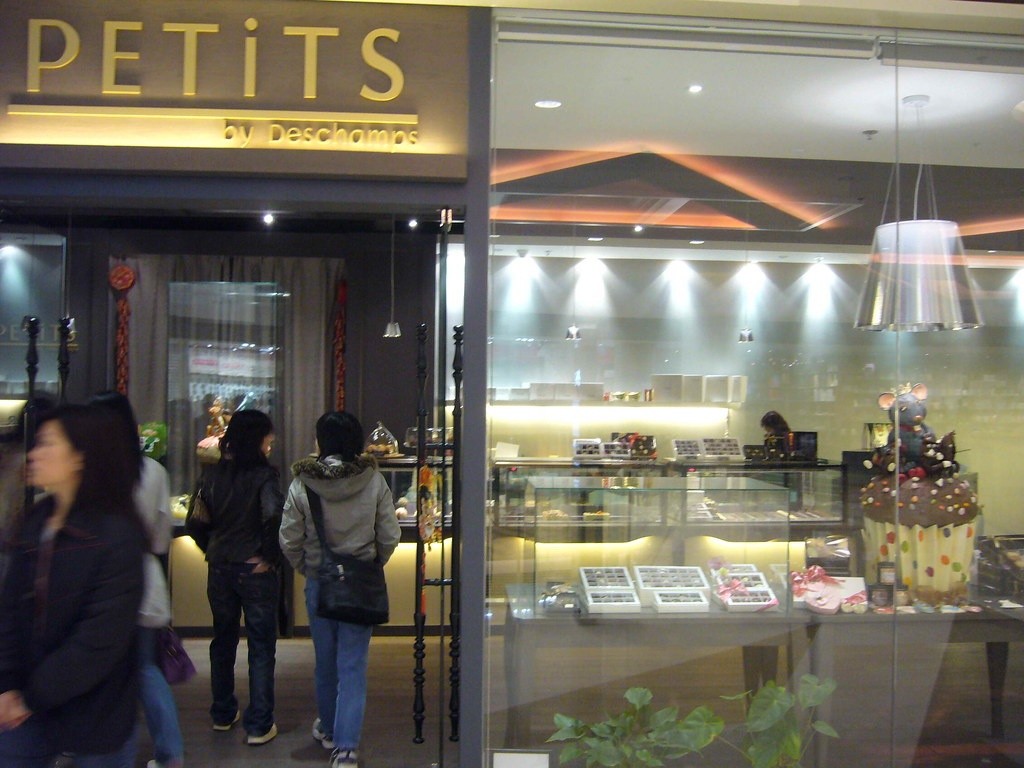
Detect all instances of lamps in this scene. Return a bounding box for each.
[565,225,583,341]
[738,231,753,342]
[383,220,401,337]
[852,93,985,333]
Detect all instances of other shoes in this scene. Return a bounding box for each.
[146,758,169,768]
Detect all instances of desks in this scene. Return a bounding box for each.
[502,580,812,767]
[757,585,1024,768]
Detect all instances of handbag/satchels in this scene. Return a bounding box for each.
[190,487,211,530]
[317,552,393,626]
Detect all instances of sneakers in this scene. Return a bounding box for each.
[247,721,277,745]
[312,717,336,749]
[330,746,357,768]
[213,708,241,730]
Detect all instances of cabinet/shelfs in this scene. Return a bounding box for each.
[494,455,852,614]
[377,456,453,543]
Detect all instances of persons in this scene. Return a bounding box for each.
[91,389,181,768]
[1,392,143,768]
[278,411,401,768]
[184,410,291,743]
[761,410,794,453]
